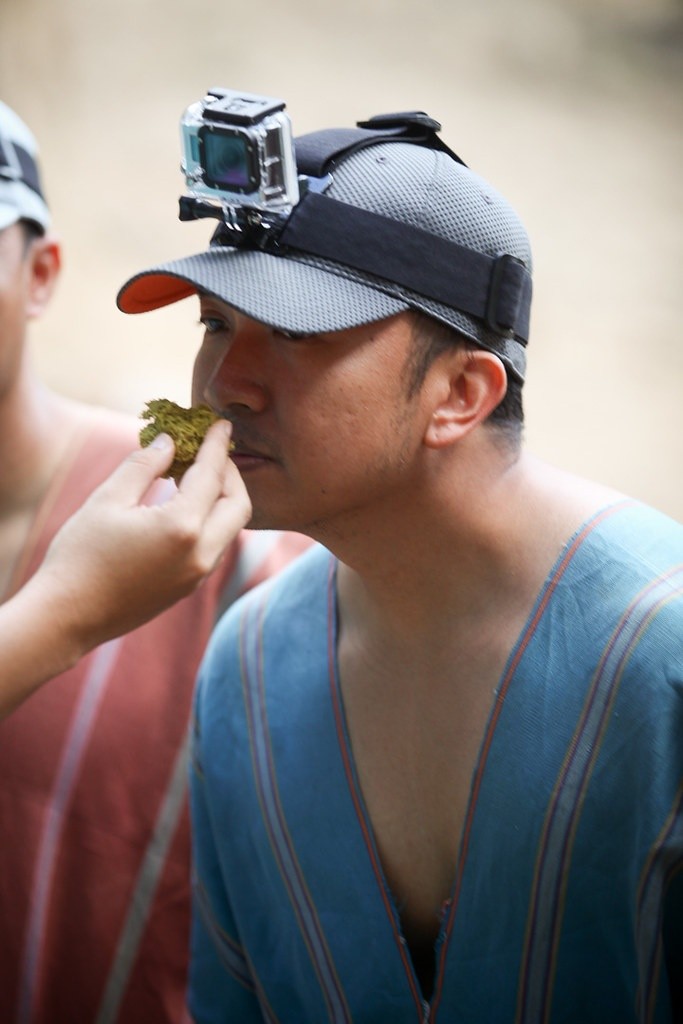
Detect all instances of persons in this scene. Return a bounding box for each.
[0,98,314,1024]
[118,111,683,1024]
[0,419,254,721]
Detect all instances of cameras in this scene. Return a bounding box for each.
[181,89,301,214]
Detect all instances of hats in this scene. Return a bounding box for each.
[117,140,533,383]
[0,101,49,234]
